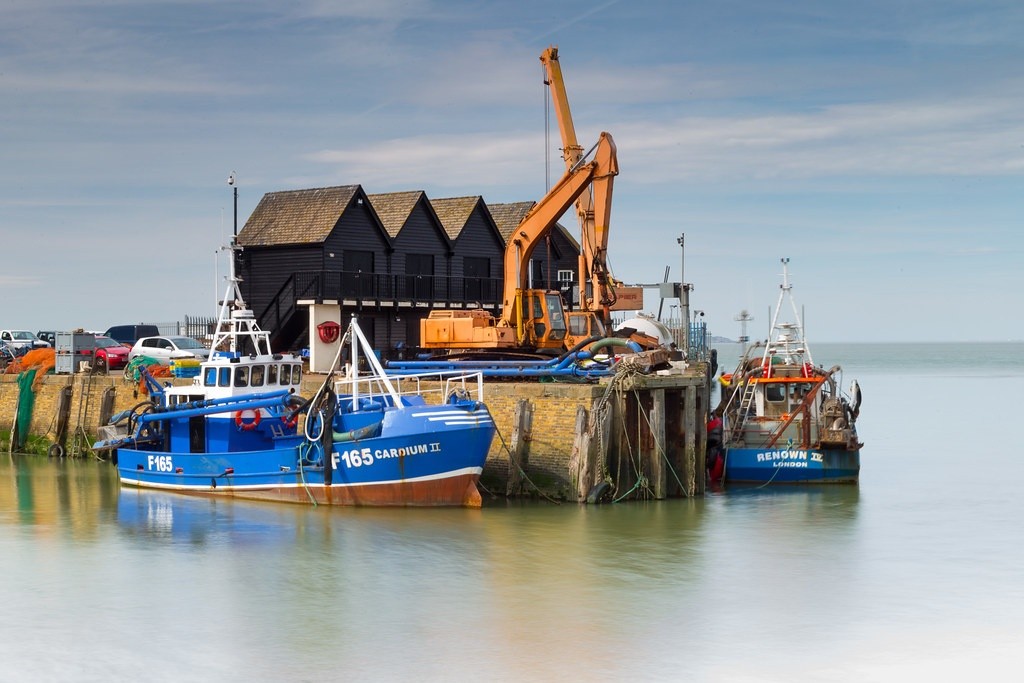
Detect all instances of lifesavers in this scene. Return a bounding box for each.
[833,417,847,431]
[282,406,299,428]
[319,321,339,343]
[235,407,261,431]
[588,481,616,505]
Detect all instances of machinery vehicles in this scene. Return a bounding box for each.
[417,131,620,352]
[538,43,615,355]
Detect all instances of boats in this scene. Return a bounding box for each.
[717,256,861,485]
[90,233,497,508]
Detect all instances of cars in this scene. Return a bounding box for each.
[80,335,131,369]
[1,330,51,355]
[37,330,55,348]
[128,335,219,367]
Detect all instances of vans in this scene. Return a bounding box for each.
[102,324,160,346]
